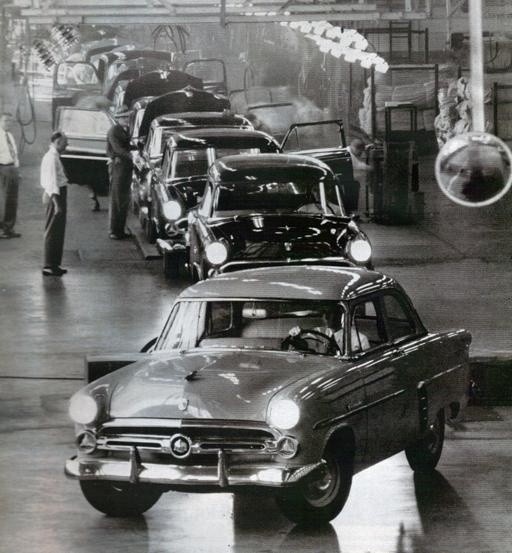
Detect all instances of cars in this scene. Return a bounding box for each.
[60,259,475,529]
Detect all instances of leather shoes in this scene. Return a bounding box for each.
[43,266,67,276]
[0,230,21,238]
[109,233,133,240]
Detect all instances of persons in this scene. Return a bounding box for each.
[102,104,146,240]
[288,302,371,355]
[38,130,72,275]
[0,110,24,238]
[245,111,273,138]
[294,181,342,217]
[342,138,376,220]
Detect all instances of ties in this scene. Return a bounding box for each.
[6,133,15,158]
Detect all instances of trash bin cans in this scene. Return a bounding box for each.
[370,147,419,214]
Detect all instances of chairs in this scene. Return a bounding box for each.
[199,317,386,351]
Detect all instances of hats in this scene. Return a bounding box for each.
[115,105,133,117]
